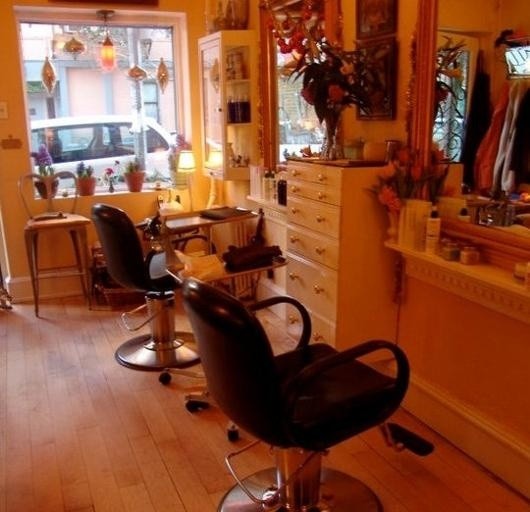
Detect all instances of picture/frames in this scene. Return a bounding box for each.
[355,1,399,122]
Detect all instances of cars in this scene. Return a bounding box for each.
[274,120,325,164]
[432,115,464,159]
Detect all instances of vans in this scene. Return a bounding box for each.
[27,108,179,193]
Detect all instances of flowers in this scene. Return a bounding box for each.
[269,4,389,127]
[433,140,460,198]
[104,159,120,181]
[20,145,77,186]
[78,162,94,177]
[430,33,469,130]
[167,134,193,168]
[366,142,424,213]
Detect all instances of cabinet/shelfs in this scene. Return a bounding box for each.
[276,155,400,360]
[197,27,263,178]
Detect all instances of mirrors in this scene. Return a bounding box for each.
[416,2,529,239]
[259,3,337,167]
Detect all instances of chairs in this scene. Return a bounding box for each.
[88,201,213,375]
[172,276,439,510]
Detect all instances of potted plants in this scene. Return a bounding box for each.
[122,157,146,194]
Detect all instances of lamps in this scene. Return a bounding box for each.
[177,150,198,175]
[92,10,117,70]
[205,149,224,171]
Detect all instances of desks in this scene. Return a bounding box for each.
[24,212,93,317]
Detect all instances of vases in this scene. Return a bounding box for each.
[388,208,403,228]
[170,167,178,185]
[33,175,60,200]
[319,114,340,159]
[108,177,116,194]
[76,175,96,196]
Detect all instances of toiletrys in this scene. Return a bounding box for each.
[511,262,527,286]
[441,243,460,261]
[459,246,480,265]
